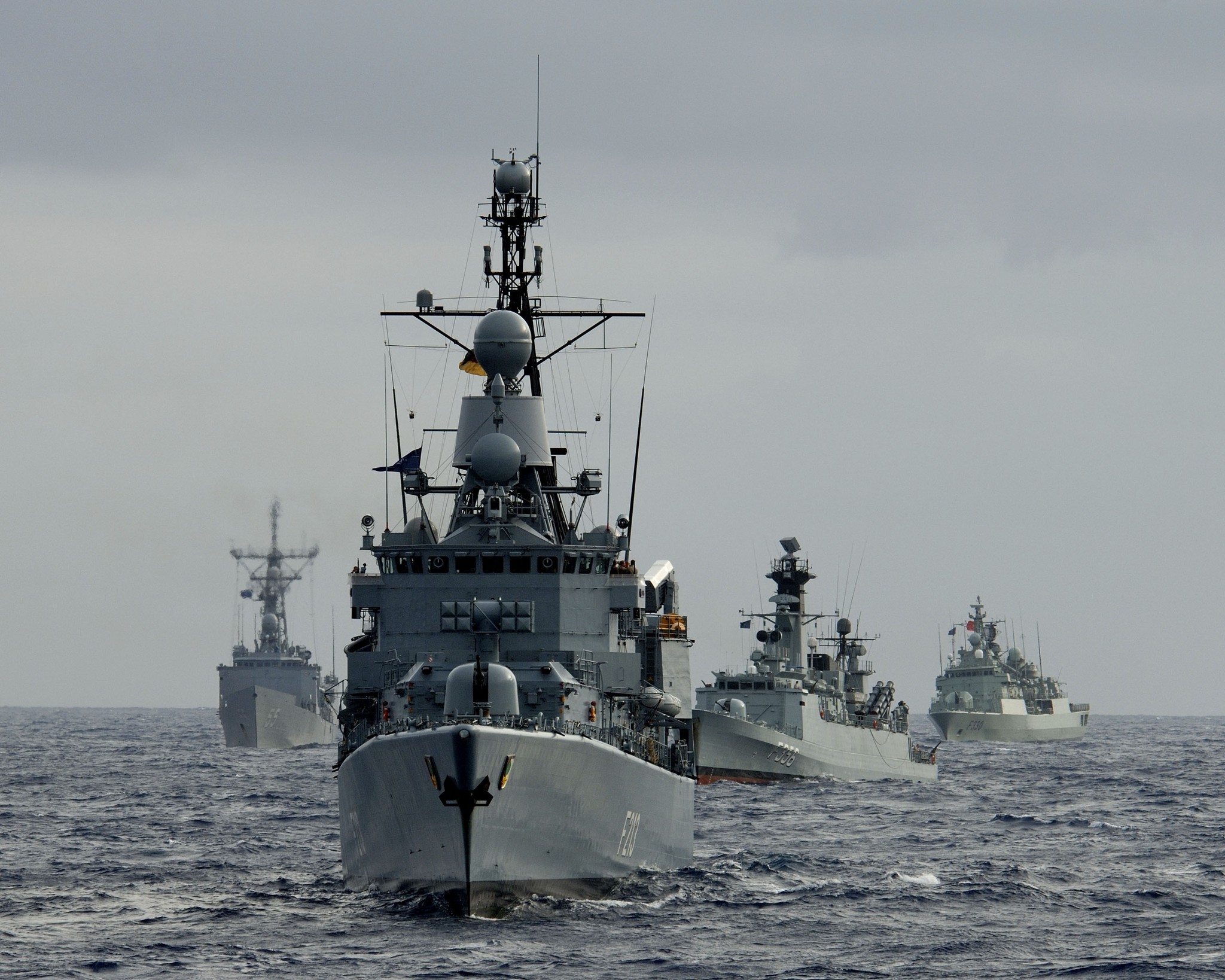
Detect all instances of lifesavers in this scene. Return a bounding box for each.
[382,706,388,721]
[590,707,596,722]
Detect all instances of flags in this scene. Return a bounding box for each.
[967,621,974,631]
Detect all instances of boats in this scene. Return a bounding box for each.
[929,595,1094,744]
[328,48,706,915]
[215,502,344,751]
[688,528,947,790]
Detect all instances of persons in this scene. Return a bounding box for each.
[610,559,638,575]
[794,681,799,689]
